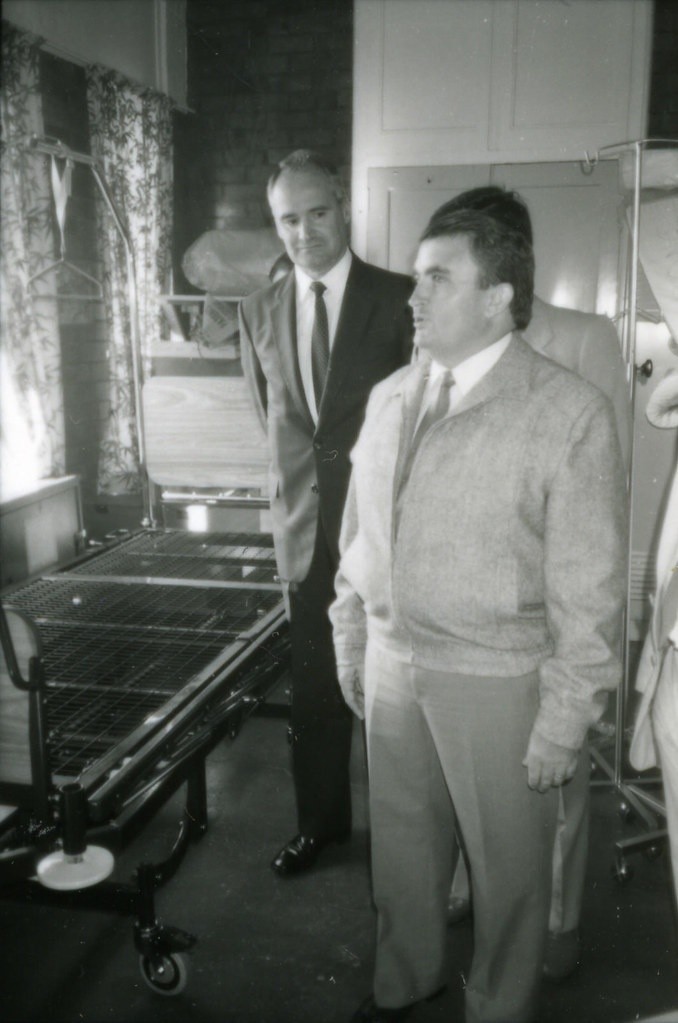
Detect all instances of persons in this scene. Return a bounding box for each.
[427,182,634,986]
[328,208,627,1022]
[238,146,416,878]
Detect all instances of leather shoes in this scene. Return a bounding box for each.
[272,833,346,875]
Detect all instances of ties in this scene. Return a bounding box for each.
[309,282,330,417]
[395,370,455,503]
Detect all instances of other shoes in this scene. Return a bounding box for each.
[351,984,448,1023]
[540,929,584,983]
[445,896,473,925]
